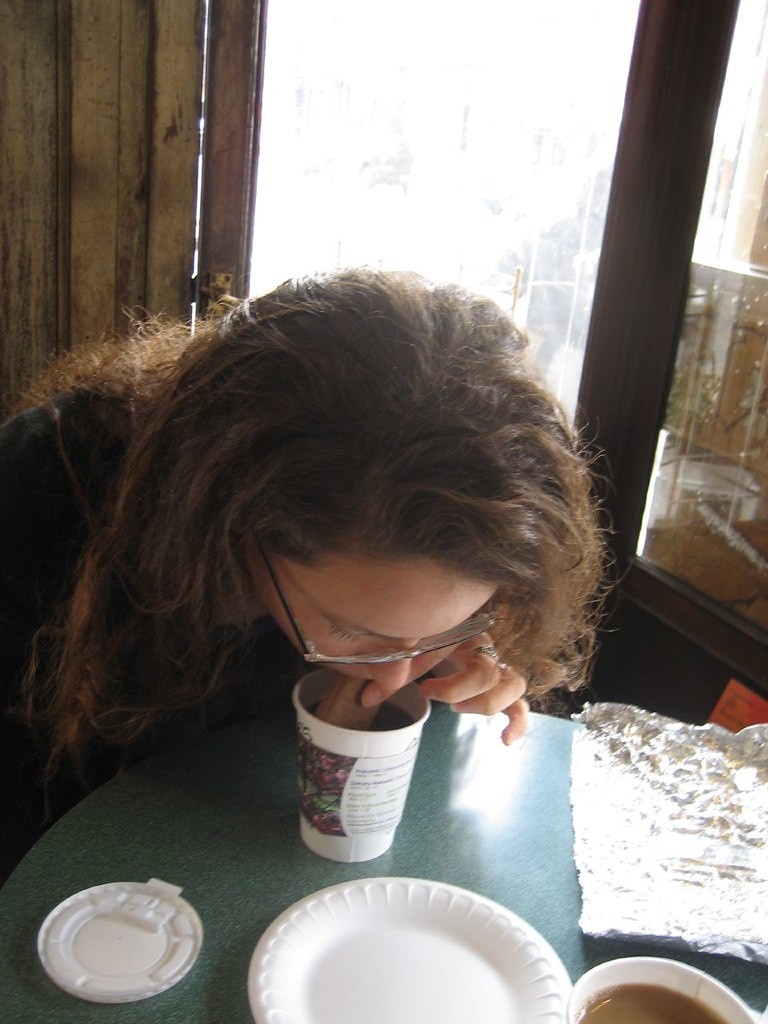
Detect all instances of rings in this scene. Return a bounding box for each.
[468,646,500,662]
[494,661,512,671]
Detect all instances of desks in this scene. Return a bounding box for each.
[0,684,765,1024]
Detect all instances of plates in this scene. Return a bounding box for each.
[247,877,572,1024]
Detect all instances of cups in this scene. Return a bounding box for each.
[292,669,430,862]
[567,957,760,1024]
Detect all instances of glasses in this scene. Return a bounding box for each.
[253,534,496,664]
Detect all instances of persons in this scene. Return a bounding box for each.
[0,268,602,888]
